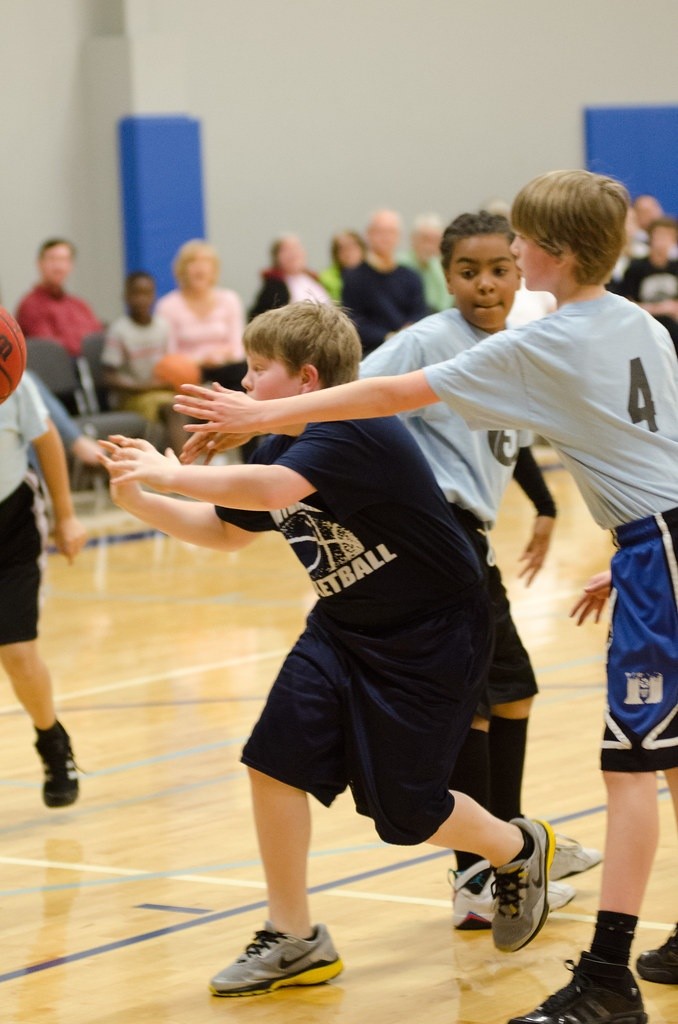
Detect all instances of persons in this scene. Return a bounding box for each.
[12,194,678,464]
[94,298,553,997]
[172,168,678,1024]
[0,372,101,807]
[179,211,603,931]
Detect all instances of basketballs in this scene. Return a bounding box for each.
[0,305,26,404]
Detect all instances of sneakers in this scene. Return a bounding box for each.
[35,719,79,808]
[506,950,647,1024]
[491,817,555,953]
[551,831,605,881]
[636,935,678,984]
[447,860,575,930]
[208,921,344,997]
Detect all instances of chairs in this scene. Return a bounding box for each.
[21,338,149,509]
[76,330,143,414]
